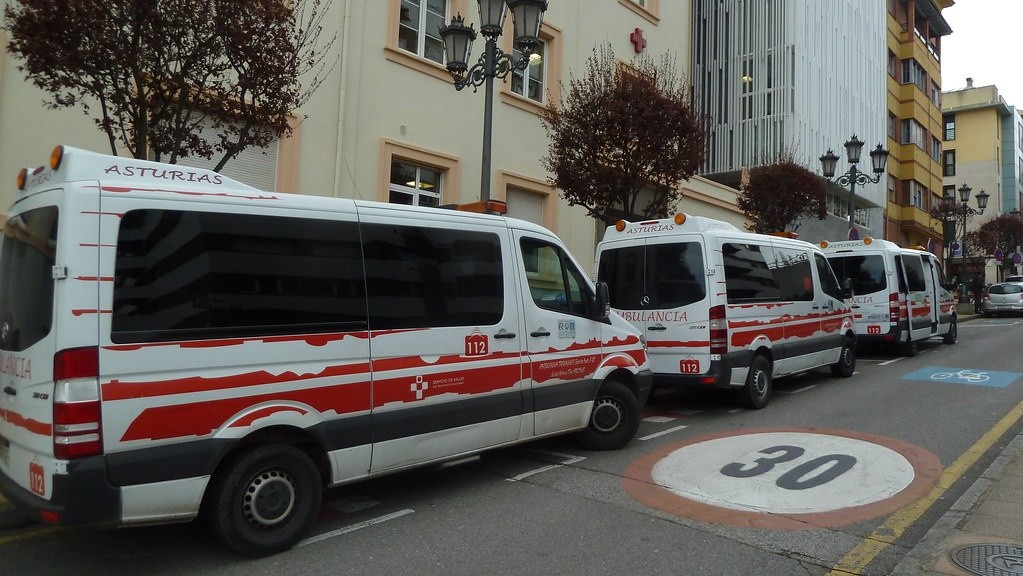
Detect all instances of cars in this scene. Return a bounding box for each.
[983,281,1023,316]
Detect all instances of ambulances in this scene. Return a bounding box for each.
[813,237,958,356]
[0,146,651,559]
[592,213,857,409]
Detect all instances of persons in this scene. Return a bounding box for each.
[974,270,986,314]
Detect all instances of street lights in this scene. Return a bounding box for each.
[437,0,547,201]
[943,181,990,314]
[818,133,890,240]
